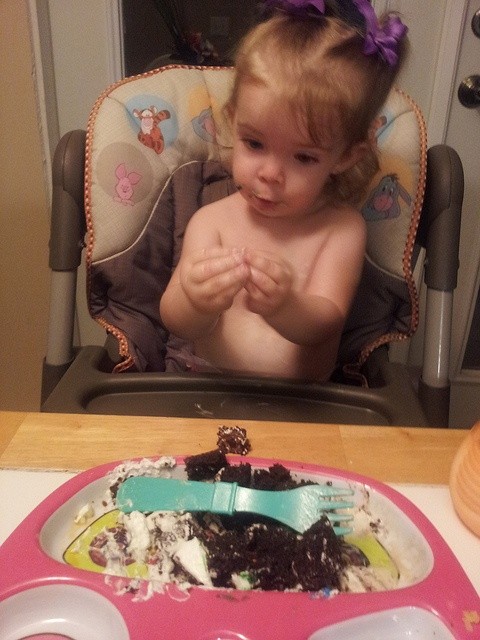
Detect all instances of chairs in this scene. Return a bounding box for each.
[40,128,466,431]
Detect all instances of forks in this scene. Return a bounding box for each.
[117,477,355,546]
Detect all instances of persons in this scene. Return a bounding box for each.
[159,0,410,384]
